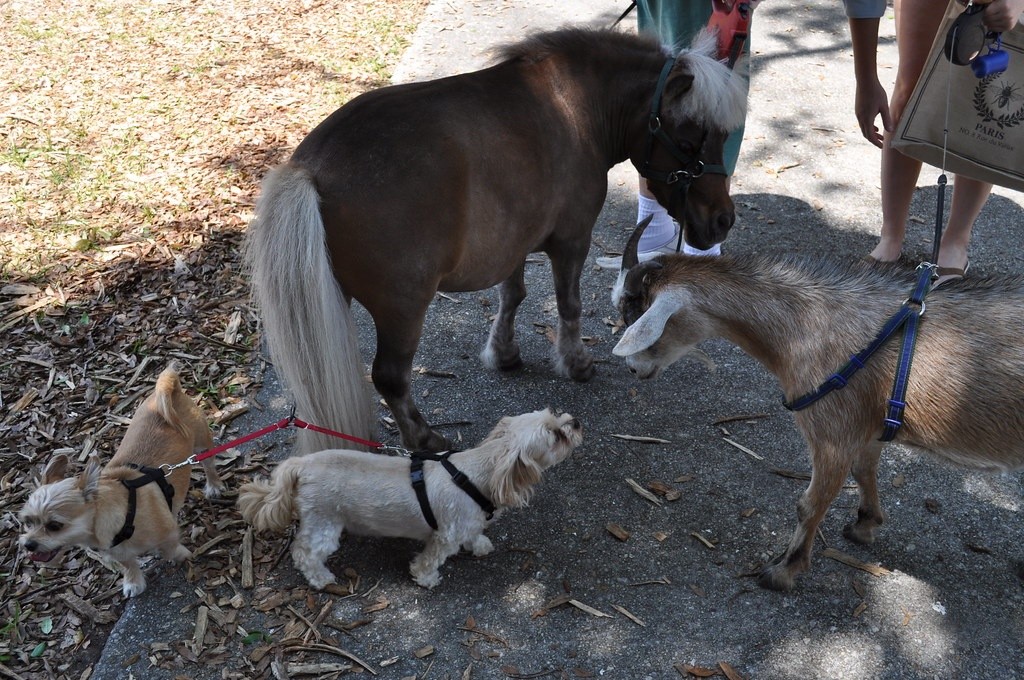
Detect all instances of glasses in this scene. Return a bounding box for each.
[945,0,1002,65]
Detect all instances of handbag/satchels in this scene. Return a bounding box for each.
[889,0,1024,193]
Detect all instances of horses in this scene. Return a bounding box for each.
[242,25,737,454]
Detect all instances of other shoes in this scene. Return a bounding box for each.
[637,222,685,263]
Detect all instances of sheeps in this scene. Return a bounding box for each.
[617,210,1024,594]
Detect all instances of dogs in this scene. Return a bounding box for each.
[18,364,220,600]
[240,405,583,591]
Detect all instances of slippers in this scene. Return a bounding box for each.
[931,261,969,283]
[856,250,902,264]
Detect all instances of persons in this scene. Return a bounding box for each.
[841,0,1024,291]
[596,0,754,271]
